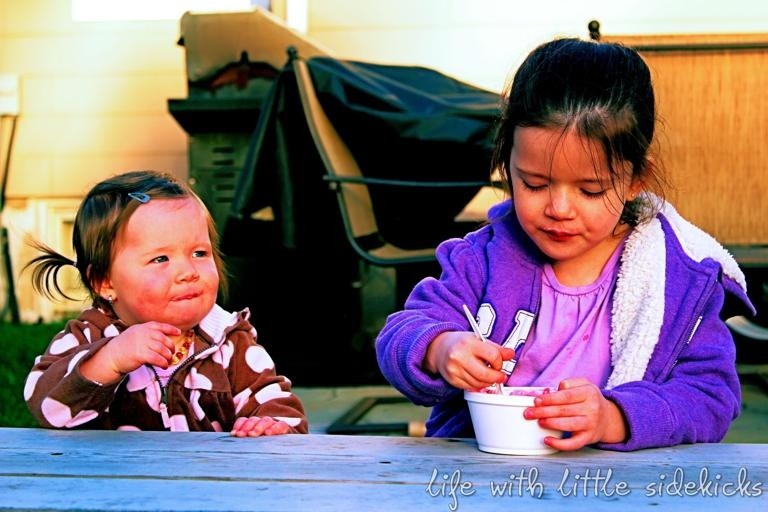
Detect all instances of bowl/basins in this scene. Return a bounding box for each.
[463,385,563,454]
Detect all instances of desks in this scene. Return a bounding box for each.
[0,422,768,511]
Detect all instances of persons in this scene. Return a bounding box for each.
[18,170,308,436]
[375,39,758,451]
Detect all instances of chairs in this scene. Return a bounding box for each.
[283,42,514,333]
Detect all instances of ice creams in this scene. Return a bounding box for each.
[473,386,564,398]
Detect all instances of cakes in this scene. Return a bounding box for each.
[165,330,194,365]
